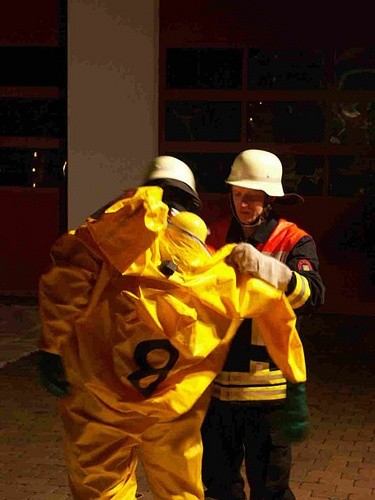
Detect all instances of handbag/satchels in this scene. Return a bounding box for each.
[272,380,310,441]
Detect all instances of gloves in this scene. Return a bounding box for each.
[37,350,72,398]
[228,242,293,292]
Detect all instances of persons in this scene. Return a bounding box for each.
[201,150,325,500]
[38,155,242,500]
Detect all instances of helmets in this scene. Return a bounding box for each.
[141,155,201,203]
[226,149,284,198]
